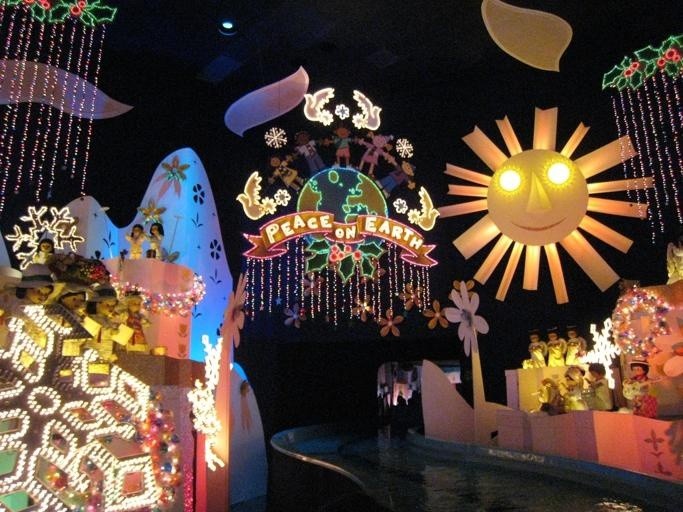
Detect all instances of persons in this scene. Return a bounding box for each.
[32,238,56,265]
[144,222,163,258]
[61,272,89,309]
[86,282,119,315]
[527,327,655,416]
[124,291,150,344]
[9,262,57,301]
[124,224,144,259]
